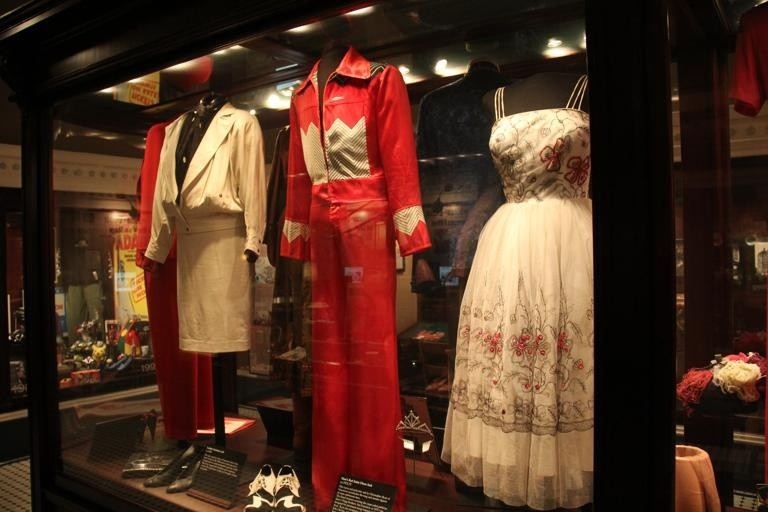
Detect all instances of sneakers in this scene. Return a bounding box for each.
[276,465,306,512]
[244,464,276,511]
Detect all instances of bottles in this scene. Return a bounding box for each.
[124,320,142,357]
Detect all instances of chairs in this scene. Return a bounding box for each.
[409,334,456,417]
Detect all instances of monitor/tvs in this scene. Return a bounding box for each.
[437,264,460,288]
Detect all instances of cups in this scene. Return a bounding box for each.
[141,345,149,357]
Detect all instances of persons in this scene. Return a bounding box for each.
[144,92,267,354]
[262,125,305,397]
[441,51,593,512]
[133,109,216,442]
[279,39,435,512]
[411,58,517,292]
[57,222,107,351]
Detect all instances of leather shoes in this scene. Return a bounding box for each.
[143,444,207,493]
